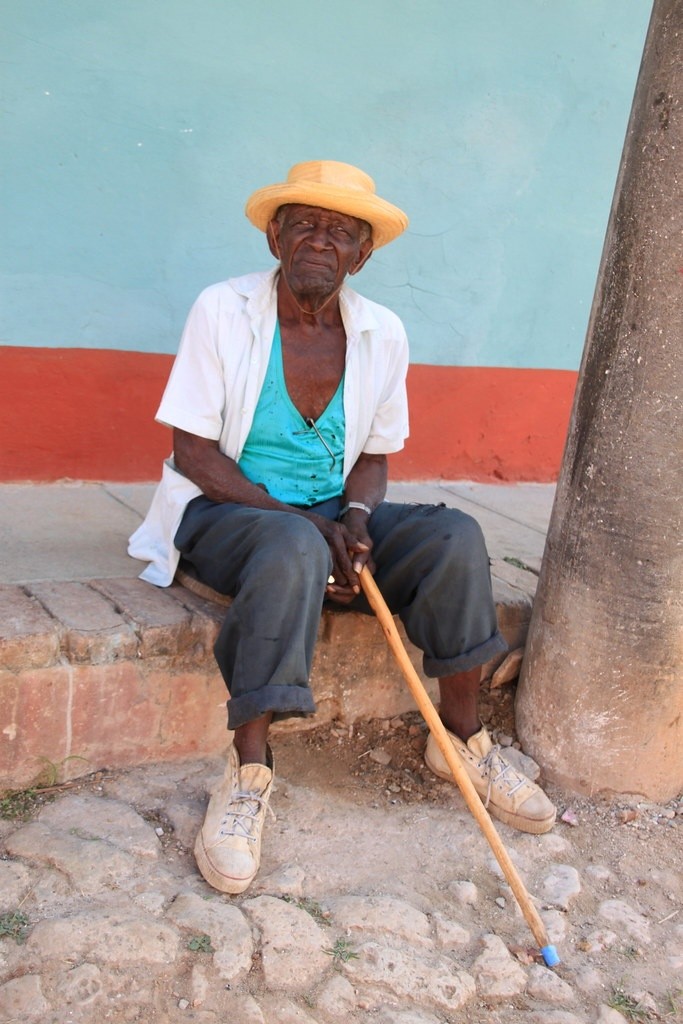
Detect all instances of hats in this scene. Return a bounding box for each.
[245,158,409,249]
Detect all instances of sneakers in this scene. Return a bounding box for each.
[192,738,278,895]
[424,721,557,834]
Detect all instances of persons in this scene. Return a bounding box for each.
[126,159,557,894]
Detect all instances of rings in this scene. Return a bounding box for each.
[328,576,335,584]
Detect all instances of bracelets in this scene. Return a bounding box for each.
[338,501,371,522]
